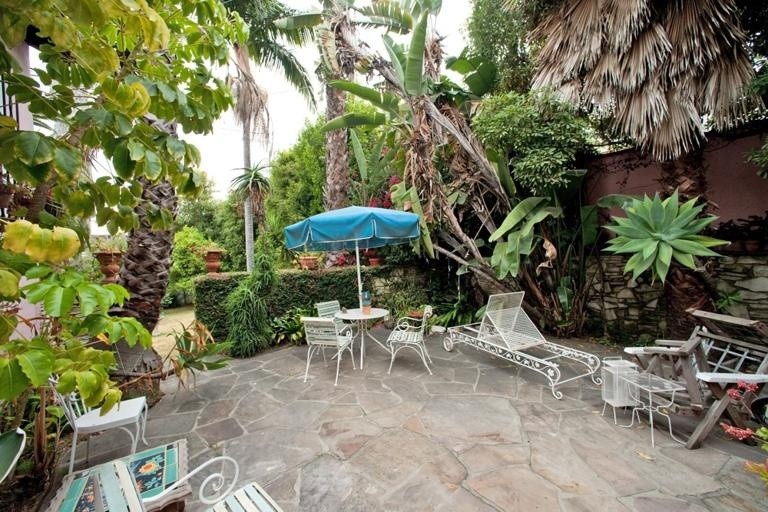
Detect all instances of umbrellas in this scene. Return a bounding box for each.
[283,205,421,358]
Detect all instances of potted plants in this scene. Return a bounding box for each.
[364,249,381,266]
[199,243,224,274]
[92,234,128,285]
[296,251,322,270]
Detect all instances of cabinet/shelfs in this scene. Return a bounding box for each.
[600,356,641,426]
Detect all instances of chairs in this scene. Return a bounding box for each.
[47,373,148,474]
[300,300,359,385]
[386,306,435,375]
[142,454,284,512]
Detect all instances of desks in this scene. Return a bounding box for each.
[619,373,686,447]
[331,308,393,370]
[46,438,193,511]
